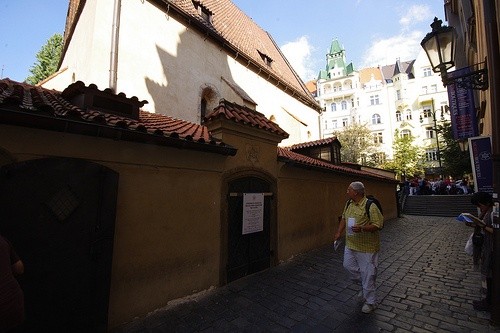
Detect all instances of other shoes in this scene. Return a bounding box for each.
[474,298,492,312]
[490,307,500,326]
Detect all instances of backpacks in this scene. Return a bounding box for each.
[346,195,385,231]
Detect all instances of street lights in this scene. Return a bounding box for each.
[418,97,445,181]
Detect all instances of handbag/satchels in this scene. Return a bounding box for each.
[472,233,483,248]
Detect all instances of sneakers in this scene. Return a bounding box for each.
[359,288,364,298]
[362,302,378,313]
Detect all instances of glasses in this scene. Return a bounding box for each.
[346,189,355,193]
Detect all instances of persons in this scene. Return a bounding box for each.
[334,182,383,315]
[407,175,500,326]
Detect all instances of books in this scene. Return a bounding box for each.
[456,212,486,226]
